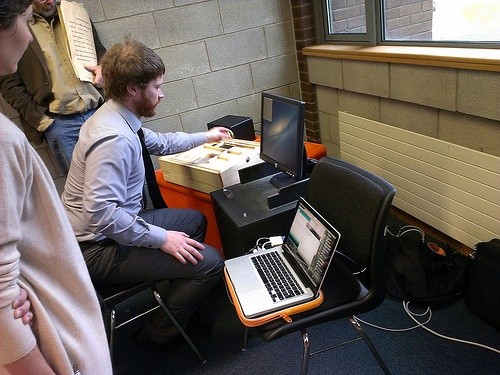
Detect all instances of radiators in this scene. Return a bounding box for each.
[339,111,500,251]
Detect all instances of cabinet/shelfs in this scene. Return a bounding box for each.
[154,136,327,261]
[210,169,307,262]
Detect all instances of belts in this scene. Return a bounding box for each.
[46,109,94,121]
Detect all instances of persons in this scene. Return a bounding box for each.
[0,0,114,375]
[61,41,230,366]
[0,0,107,186]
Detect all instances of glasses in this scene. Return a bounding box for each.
[35,0,48,4]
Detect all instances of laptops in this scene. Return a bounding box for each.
[225,197,340,319]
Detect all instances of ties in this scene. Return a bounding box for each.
[136,127,167,208]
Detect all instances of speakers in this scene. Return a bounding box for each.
[207,115,256,141]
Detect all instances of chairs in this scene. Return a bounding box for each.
[234,157,396,375]
[93,276,206,367]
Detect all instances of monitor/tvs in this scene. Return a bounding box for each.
[259,92,309,188]
